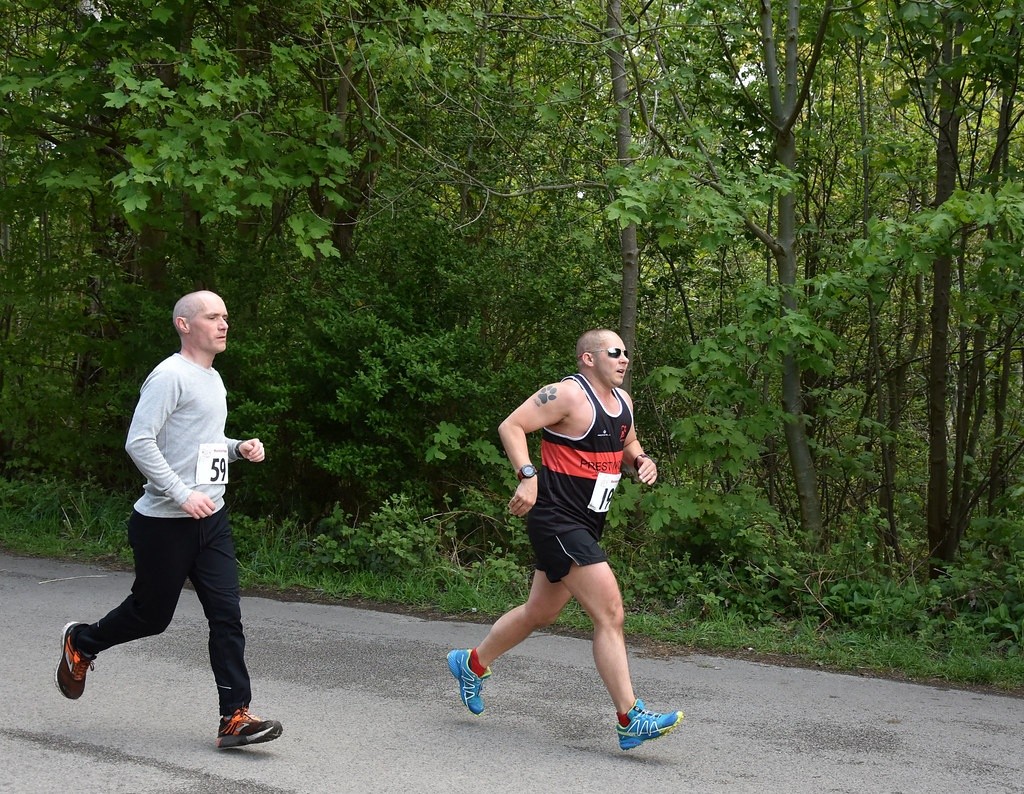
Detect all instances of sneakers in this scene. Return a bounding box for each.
[446,648,492,715]
[616,699,683,751]
[216,704,282,748]
[53,622,98,700]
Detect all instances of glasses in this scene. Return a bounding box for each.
[577,348,628,359]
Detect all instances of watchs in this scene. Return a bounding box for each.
[517,465,538,482]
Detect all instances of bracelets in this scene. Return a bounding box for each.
[235,442,244,458]
[634,454,649,471]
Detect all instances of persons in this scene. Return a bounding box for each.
[53,290,283,750]
[446,329,683,750]
